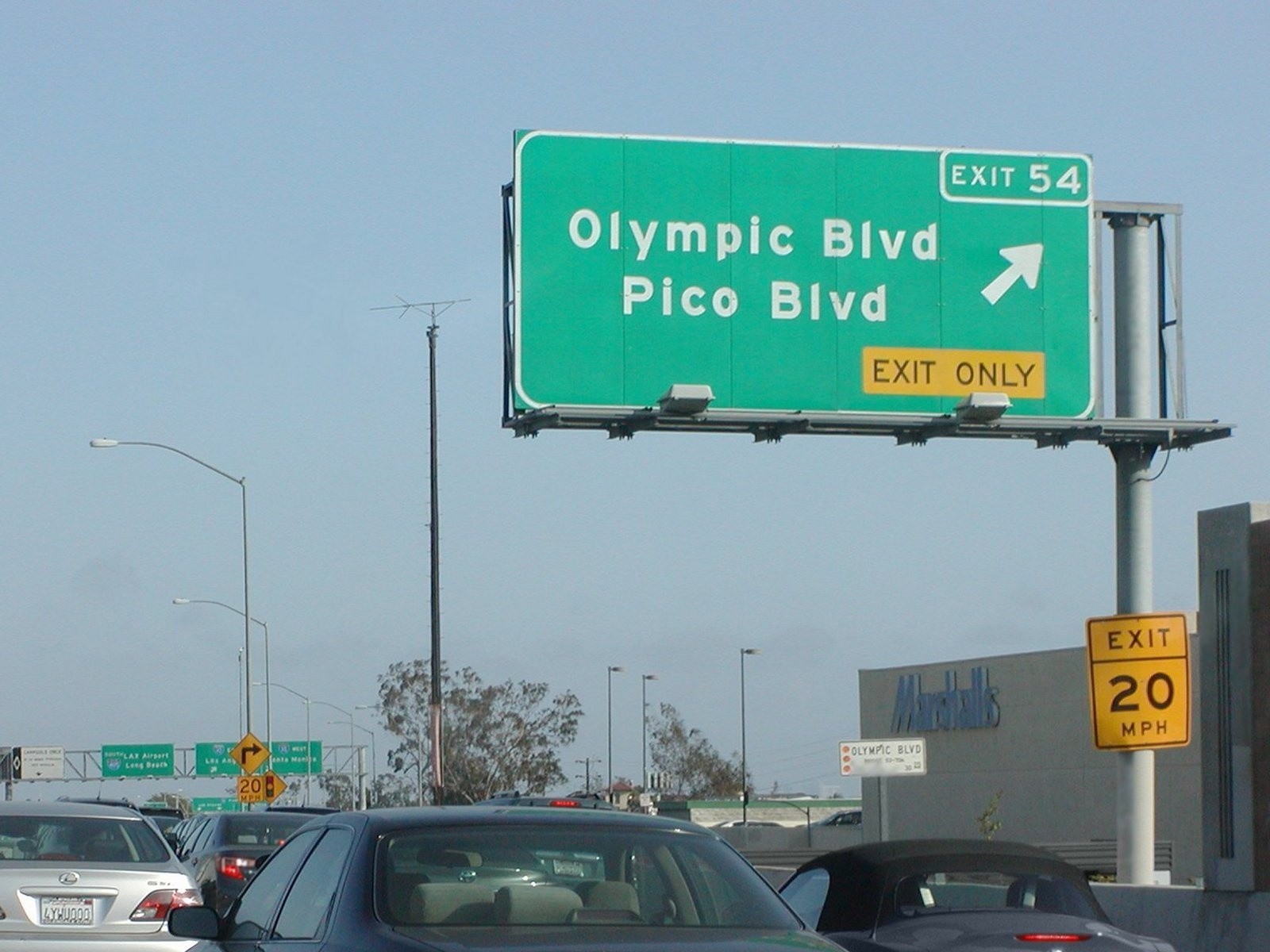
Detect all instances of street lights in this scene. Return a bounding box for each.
[251,679,315,806]
[91,436,249,788]
[302,697,359,814]
[328,718,380,806]
[743,646,757,820]
[605,662,619,797]
[641,671,655,792]
[355,702,424,806]
[173,595,274,772]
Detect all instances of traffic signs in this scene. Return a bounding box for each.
[499,128,1099,444]
[100,742,173,779]
[195,738,324,778]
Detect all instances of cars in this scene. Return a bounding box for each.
[179,799,839,952]
[1,801,219,952]
[174,808,315,919]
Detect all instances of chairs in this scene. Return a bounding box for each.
[1008,876,1056,912]
[85,838,130,863]
[235,833,259,845]
[898,878,933,917]
[409,881,638,924]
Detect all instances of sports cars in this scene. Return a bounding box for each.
[777,839,1173,951]
